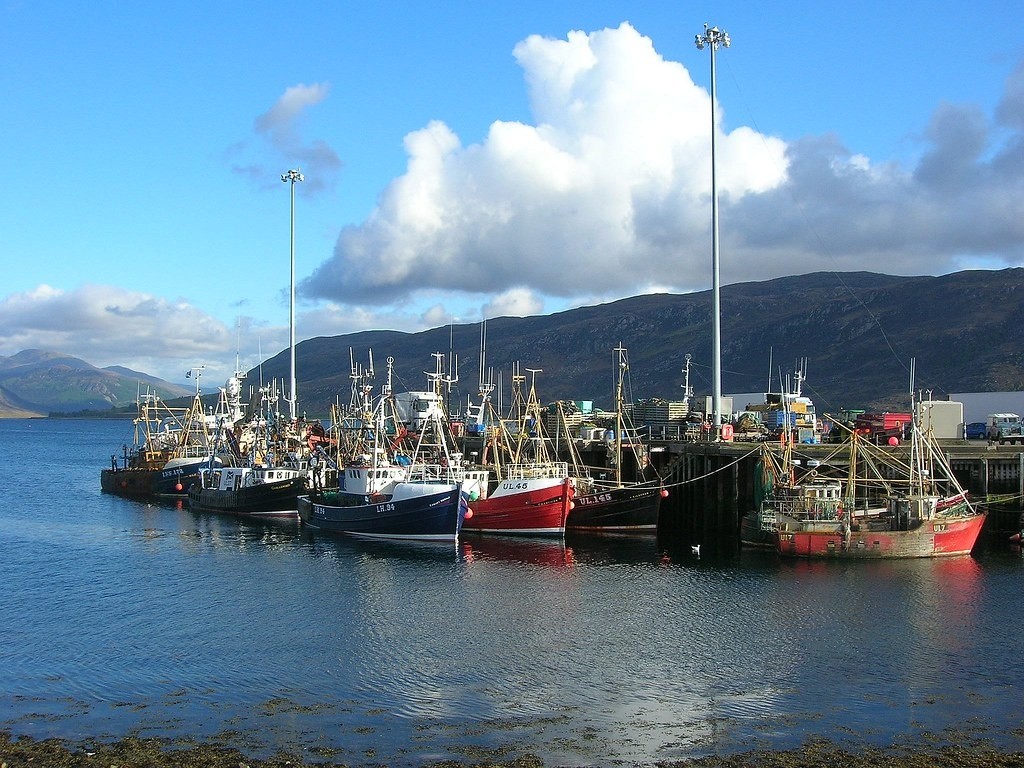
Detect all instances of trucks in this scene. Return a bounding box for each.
[722,393,818,435]
[985,413,1024,445]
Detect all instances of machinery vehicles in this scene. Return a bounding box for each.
[825,407,912,446]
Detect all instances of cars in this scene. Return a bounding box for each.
[964,422,987,440]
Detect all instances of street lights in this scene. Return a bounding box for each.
[693,22,733,442]
[281,168,305,419]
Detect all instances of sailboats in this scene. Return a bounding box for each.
[98,304,1024,561]
